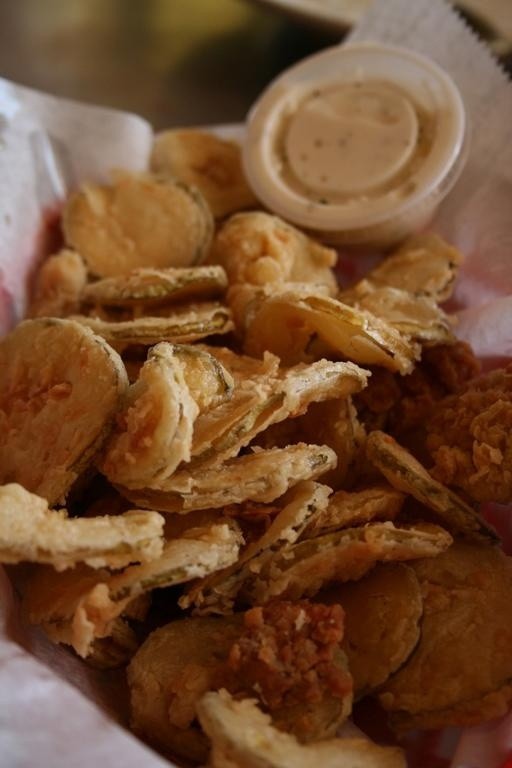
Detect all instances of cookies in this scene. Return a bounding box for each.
[1,132,511,768]
